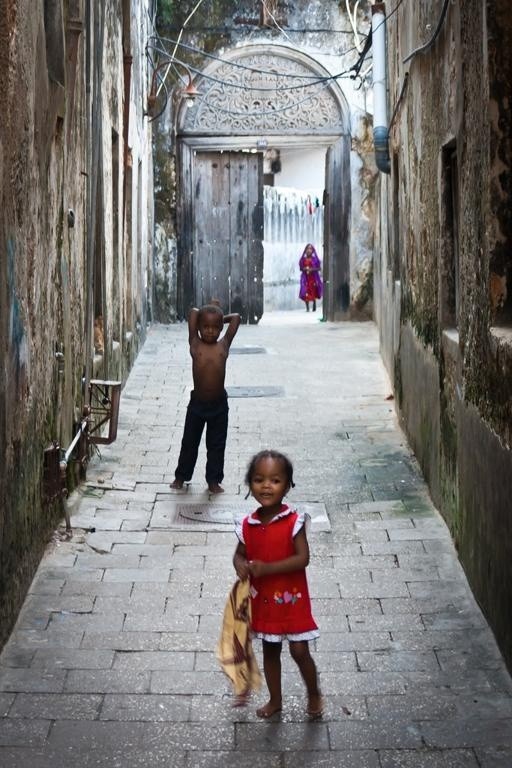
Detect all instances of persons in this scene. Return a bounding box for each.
[169,304,242,493]
[233,450,324,718]
[298,242,322,312]
